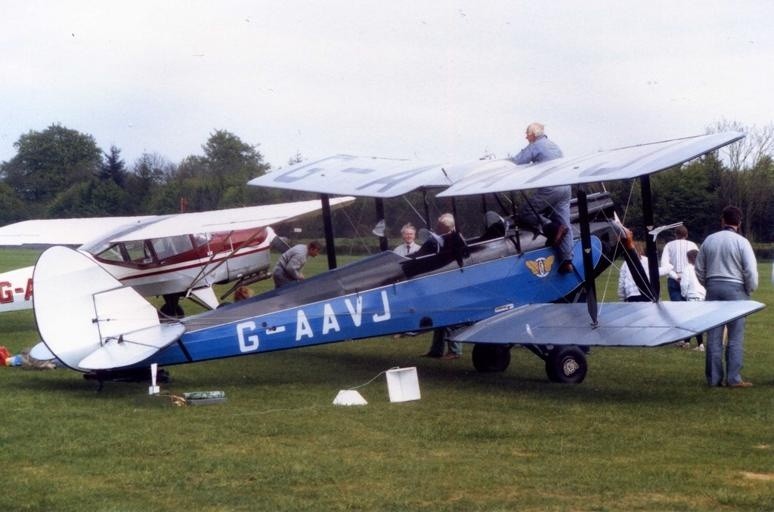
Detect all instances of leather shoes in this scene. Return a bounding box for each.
[419,350,443,358]
[440,351,461,361]
[553,225,568,244]
[731,381,753,388]
[563,262,573,272]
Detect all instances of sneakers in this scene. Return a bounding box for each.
[681,343,691,348]
[698,344,705,352]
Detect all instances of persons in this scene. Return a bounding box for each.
[390,221,422,338]
[501,122,573,273]
[615,238,673,302]
[676,250,707,351]
[694,204,759,387]
[417,213,466,359]
[271,239,323,289]
[661,225,700,347]
[232,286,254,303]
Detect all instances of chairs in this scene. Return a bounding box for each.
[439,231,466,248]
[161,237,177,257]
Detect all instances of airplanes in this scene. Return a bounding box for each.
[0,195,359,325]
[0,127,771,383]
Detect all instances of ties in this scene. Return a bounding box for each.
[406,246,410,254]
